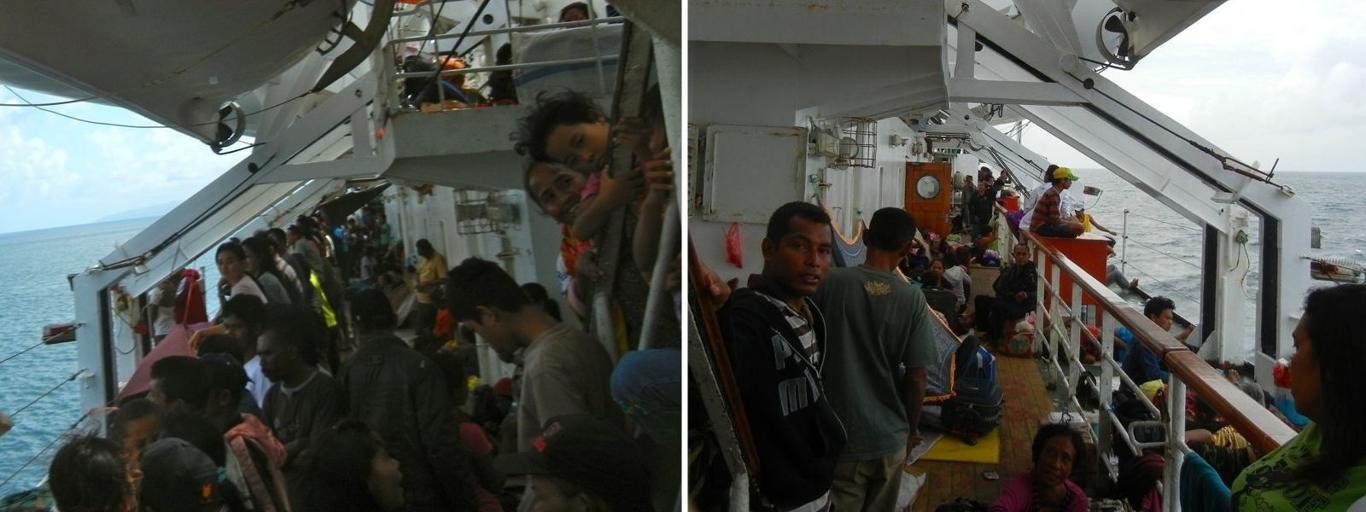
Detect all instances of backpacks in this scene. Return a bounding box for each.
[954,332,996,398]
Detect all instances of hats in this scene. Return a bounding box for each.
[199,351,249,391]
[1073,202,1085,212]
[1053,168,1077,182]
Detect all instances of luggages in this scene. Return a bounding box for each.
[939,384,1006,435]
